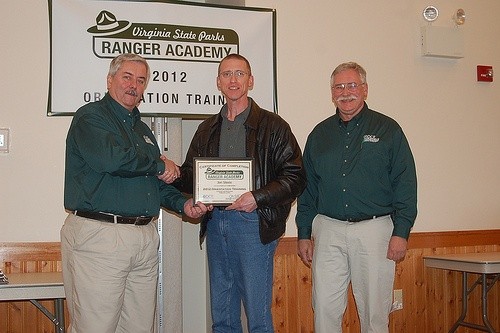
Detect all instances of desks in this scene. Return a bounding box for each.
[422,252,500,333]
[0,271,65,333]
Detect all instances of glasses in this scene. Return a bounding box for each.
[219,71,250,78]
[332,82,366,91]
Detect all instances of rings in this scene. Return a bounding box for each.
[199,212,204,215]
[174,175,177,179]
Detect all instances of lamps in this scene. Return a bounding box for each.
[422,6,466,57]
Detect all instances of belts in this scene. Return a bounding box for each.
[72,208,159,226]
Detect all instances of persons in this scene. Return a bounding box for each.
[60,54,214,333]
[296,62,417,333]
[158,53,307,333]
